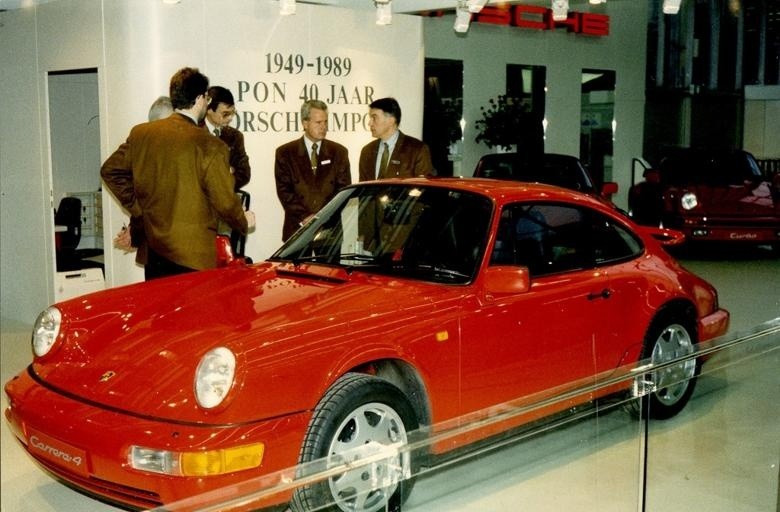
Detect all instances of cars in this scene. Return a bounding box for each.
[4,177,732,512]
[473,153,598,195]
[628,148,780,246]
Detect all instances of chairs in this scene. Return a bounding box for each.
[400,205,619,284]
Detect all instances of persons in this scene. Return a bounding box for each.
[101,67,251,281]
[275,98,433,265]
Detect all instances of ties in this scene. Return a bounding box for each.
[310,143,319,177]
[213,129,220,138]
[378,142,389,180]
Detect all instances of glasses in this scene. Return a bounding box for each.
[203,94,212,105]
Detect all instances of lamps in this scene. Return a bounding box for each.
[278,0,685,33]
